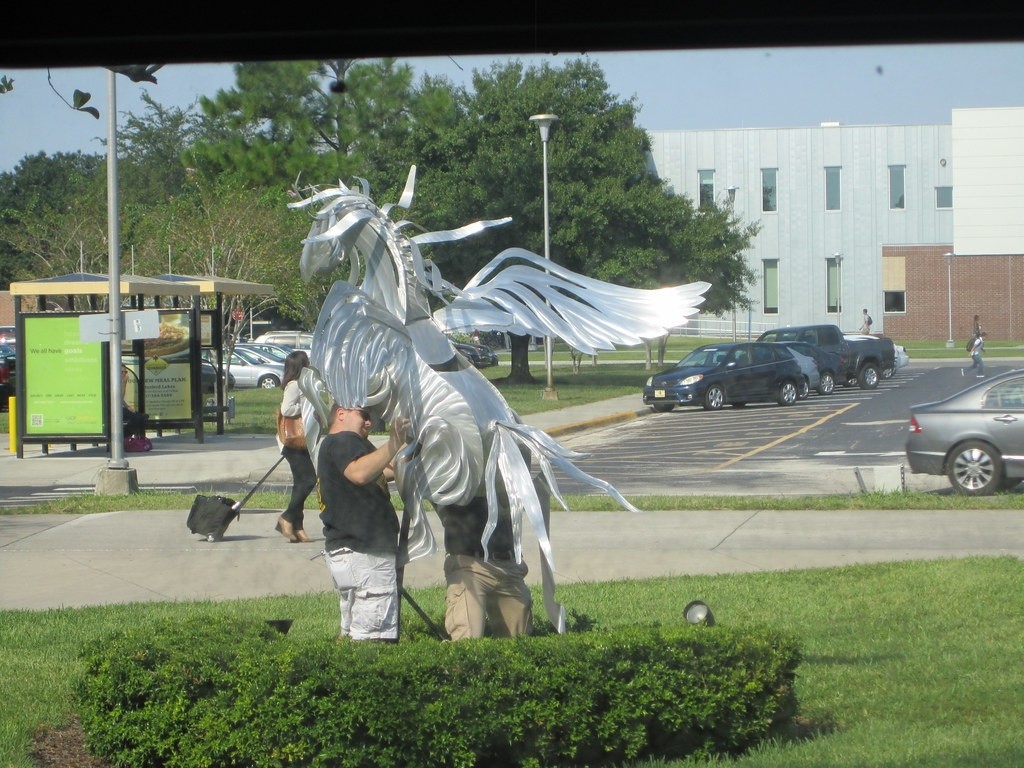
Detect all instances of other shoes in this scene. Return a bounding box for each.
[961,369,964,376]
[976,375,984,378]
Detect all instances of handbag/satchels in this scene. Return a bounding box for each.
[276,408,321,449]
[865,315,872,325]
[125,434,153,452]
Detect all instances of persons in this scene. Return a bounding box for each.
[429,494,534,641]
[859,309,871,335]
[120,363,148,438]
[318,401,411,645]
[275,351,318,543]
[972,315,984,351]
[961,332,986,378]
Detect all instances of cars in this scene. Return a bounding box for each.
[776,342,848,396]
[234,343,293,360]
[843,334,910,382]
[209,347,287,390]
[904,369,1024,496]
[0,325,18,404]
[121,352,235,396]
[445,334,499,369]
[642,342,805,411]
[697,345,820,400]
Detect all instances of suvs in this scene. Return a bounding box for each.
[256,329,314,361]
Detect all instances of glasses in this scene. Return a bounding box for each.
[122,371,127,373]
[347,408,370,421]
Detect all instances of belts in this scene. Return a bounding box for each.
[450,550,515,560]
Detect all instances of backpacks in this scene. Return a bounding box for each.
[966,338,981,352]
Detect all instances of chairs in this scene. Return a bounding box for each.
[716,354,726,362]
[735,354,748,368]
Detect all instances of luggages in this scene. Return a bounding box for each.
[186,455,286,544]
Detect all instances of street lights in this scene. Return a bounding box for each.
[529,114,562,402]
[943,252,955,348]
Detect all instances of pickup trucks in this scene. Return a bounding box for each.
[757,324,896,391]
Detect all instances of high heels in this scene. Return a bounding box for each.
[290,530,315,543]
[275,516,297,540]
